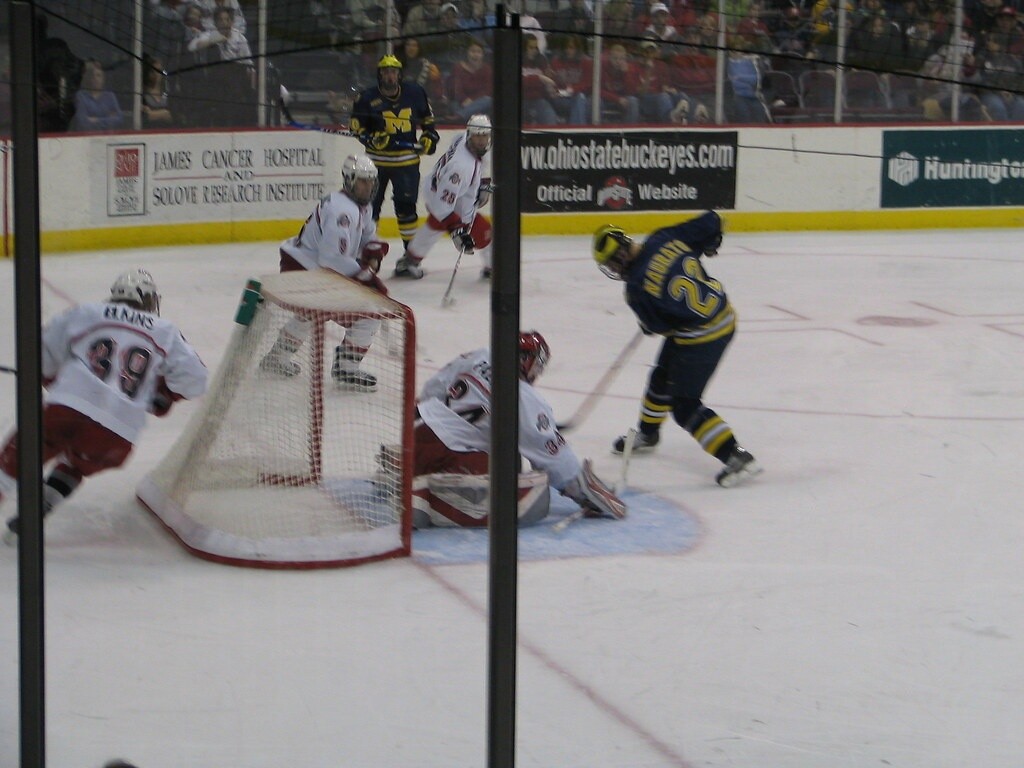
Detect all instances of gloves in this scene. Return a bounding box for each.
[475,185,492,208]
[449,226,475,256]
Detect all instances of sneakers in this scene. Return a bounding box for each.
[395,251,425,279]
[713,433,759,487]
[482,265,492,278]
[260,336,300,376]
[330,346,380,393]
[5,501,53,550]
[612,420,660,454]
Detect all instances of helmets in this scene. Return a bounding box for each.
[466,113,491,158]
[591,224,631,281]
[377,54,404,90]
[519,331,552,387]
[109,266,161,317]
[342,152,379,204]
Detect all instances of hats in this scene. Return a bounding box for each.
[639,36,658,49]
[438,1,458,14]
[650,2,669,15]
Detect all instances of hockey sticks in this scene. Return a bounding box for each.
[552,427,636,532]
[555,329,644,431]
[0,365,16,374]
[442,200,481,308]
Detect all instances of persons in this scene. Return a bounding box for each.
[22,15,86,132]
[0,268,208,535]
[349,55,440,280]
[393,113,494,281]
[592,212,765,488]
[380,330,626,531]
[259,154,389,388]
[141,0,1024,129]
[72,59,125,130]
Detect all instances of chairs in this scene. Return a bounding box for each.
[0,0,1024,131]
